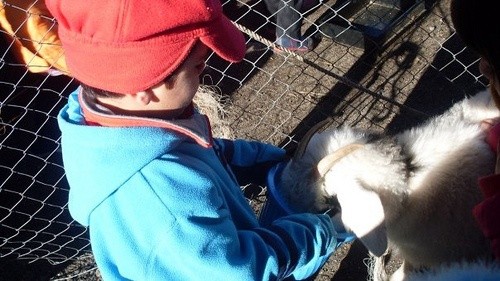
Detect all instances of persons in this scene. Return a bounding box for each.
[45,1,354,281]
[448,0,500,263]
[264,0,315,55]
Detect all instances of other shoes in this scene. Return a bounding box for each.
[272,33,313,54]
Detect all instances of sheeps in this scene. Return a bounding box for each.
[278,86,500,281]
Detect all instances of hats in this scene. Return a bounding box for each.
[44,0,246,94]
